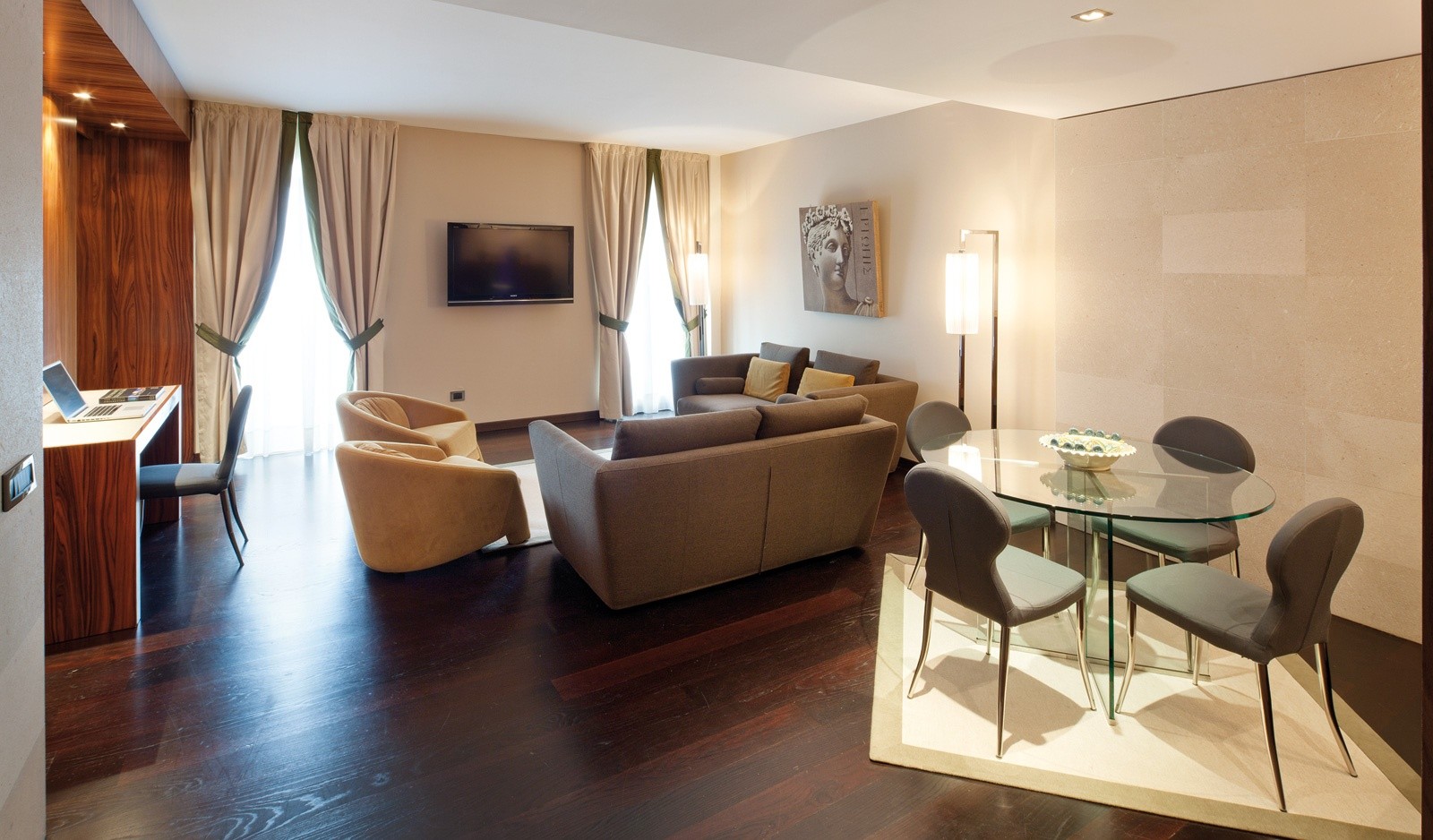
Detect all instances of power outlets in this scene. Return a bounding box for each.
[451,390,465,401]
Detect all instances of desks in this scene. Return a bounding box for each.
[44,385,188,655]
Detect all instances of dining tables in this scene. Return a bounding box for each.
[917,427,1274,683]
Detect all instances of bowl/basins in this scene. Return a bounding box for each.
[1038,430,1137,471]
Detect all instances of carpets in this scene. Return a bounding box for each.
[864,548,1421,839]
[480,448,616,554]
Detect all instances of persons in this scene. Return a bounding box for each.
[801,204,879,318]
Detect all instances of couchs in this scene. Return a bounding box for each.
[335,440,532,574]
[520,395,897,609]
[668,342,917,479]
[335,390,485,462]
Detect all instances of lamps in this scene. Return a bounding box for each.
[686,241,712,356]
[943,226,998,431]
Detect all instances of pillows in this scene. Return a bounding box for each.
[696,377,745,393]
[796,366,855,399]
[741,356,791,401]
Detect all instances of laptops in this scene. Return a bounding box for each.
[43,360,157,423]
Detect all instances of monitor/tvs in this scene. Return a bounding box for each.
[446,221,575,305]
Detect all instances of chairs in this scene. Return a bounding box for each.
[904,401,1055,589]
[1115,496,1365,815]
[139,385,252,568]
[1093,417,1257,612]
[905,464,1098,758]
